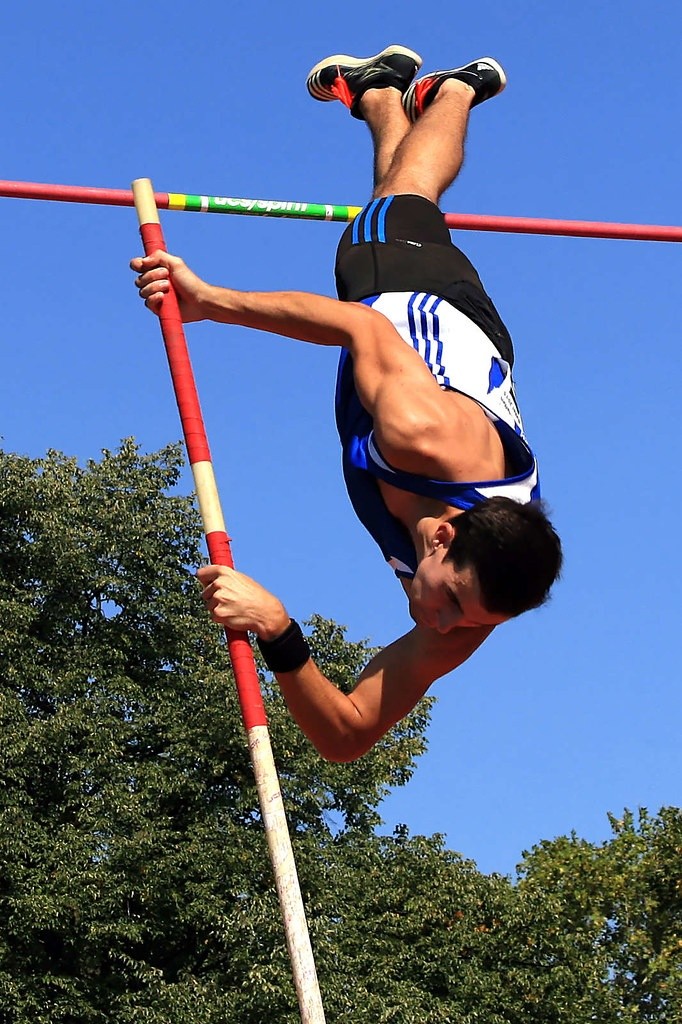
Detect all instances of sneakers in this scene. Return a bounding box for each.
[304,44,421,121]
[401,57,507,125]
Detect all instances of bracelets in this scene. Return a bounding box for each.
[256,618,311,674]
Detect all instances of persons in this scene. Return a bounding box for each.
[129,45,571,764]
